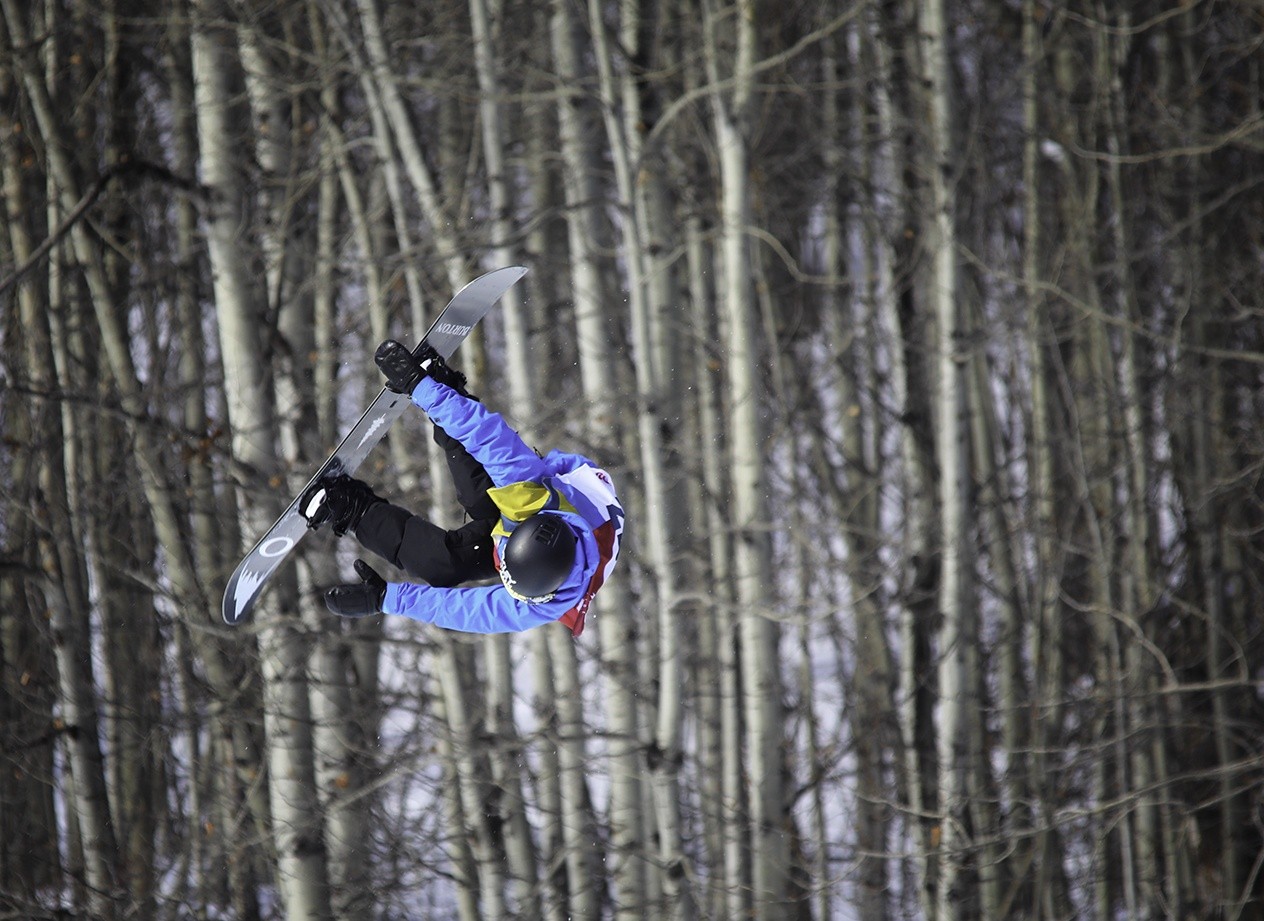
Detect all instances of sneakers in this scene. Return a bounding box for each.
[297,473,389,538]
[412,341,467,391]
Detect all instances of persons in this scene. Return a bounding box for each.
[298,339,625,637]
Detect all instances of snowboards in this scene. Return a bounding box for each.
[222,263,529,628]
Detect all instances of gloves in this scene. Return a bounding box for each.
[323,558,387,618]
[374,340,428,399]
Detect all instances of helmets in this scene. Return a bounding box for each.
[504,512,577,597]
